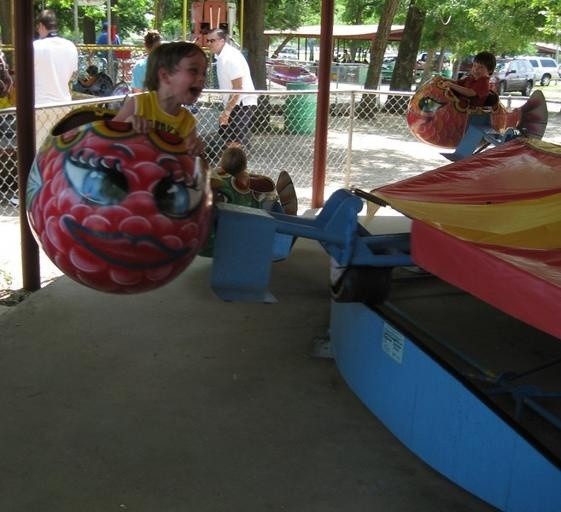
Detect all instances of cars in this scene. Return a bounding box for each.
[266,61,318,88]
[382,52,561,97]
[280,47,299,60]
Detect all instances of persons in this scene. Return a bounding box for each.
[87,22,123,86]
[218,141,256,189]
[108,40,205,158]
[31,9,80,157]
[340,49,352,63]
[1,48,16,101]
[129,30,163,96]
[78,66,100,88]
[205,28,260,160]
[440,53,500,106]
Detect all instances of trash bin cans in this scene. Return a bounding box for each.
[285,83,318,135]
[358,63,368,86]
[442,67,450,78]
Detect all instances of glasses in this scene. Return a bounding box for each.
[206,38,221,43]
